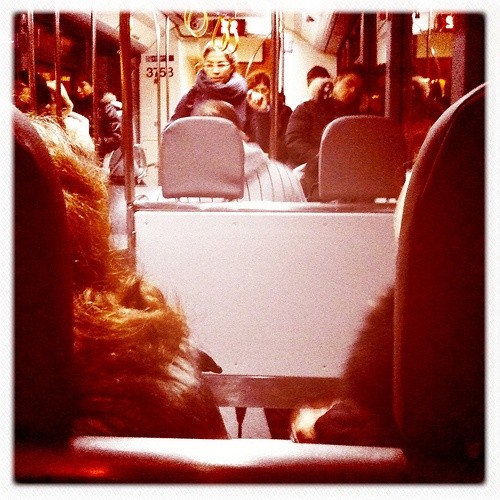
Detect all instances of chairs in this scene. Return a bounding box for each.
[318,115,408,205]
[159,116,246,204]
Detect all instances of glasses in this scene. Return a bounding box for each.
[201,62,231,71]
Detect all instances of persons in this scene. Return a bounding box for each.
[287,286,397,445]
[53,150,227,439]
[412,75,444,122]
[172,40,269,152]
[245,70,293,129]
[157,97,307,202]
[12,66,146,185]
[307,65,333,100]
[283,62,366,188]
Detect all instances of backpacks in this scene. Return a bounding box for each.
[107,146,148,181]
